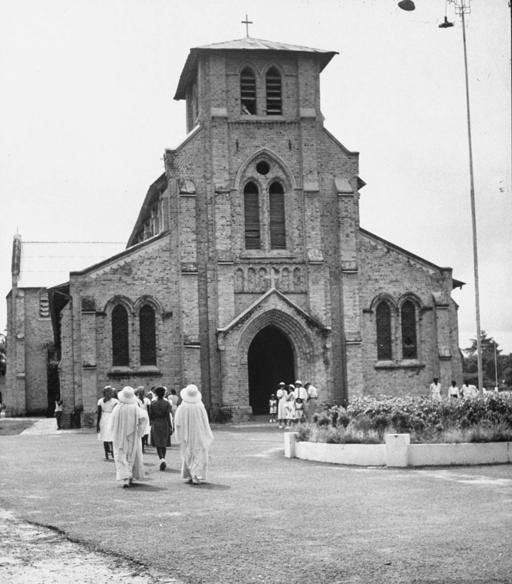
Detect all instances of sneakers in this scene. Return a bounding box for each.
[160,457,167,471]
[123,479,130,488]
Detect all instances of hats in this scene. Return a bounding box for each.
[180,384,202,404]
[117,386,139,404]
[278,380,311,392]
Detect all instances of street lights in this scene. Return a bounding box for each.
[394,0,483,391]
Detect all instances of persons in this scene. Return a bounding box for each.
[428,376,476,399]
[266,377,321,430]
[95,381,212,488]
